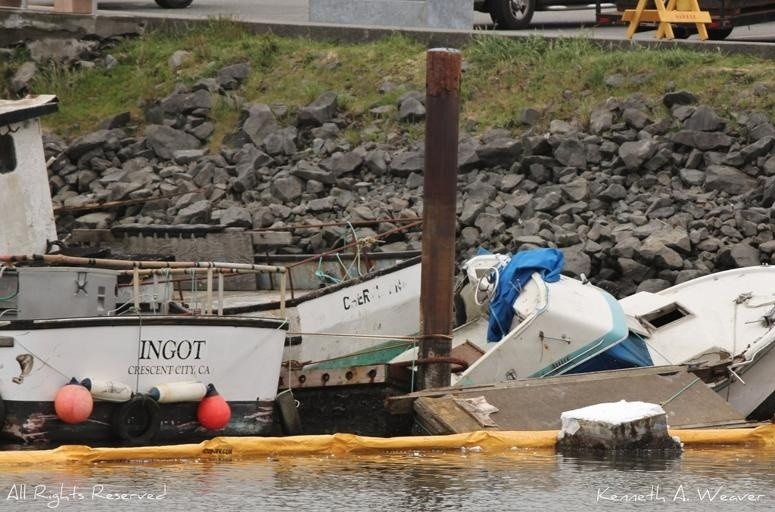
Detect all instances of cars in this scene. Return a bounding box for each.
[471,0,775,41]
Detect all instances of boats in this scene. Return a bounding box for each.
[0,90,775,454]
